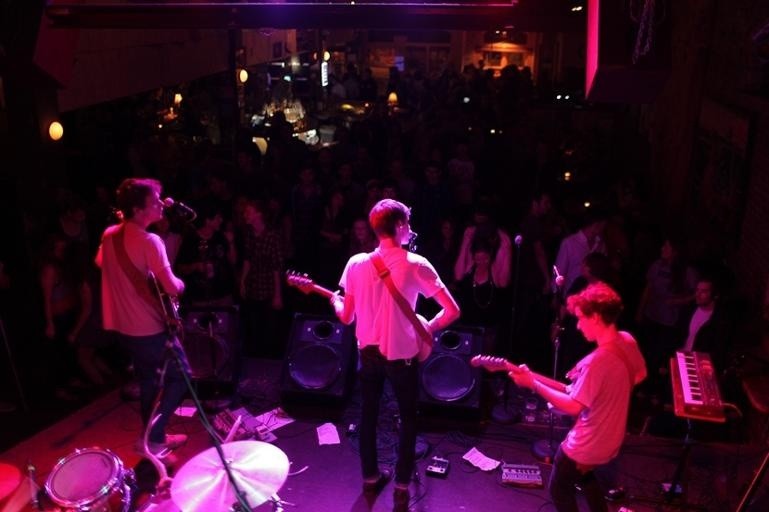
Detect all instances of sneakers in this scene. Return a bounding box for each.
[361,469,388,492]
[134,436,177,466]
[393,485,411,504]
[57,360,114,401]
[159,434,190,447]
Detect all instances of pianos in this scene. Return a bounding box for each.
[675,348,723,414]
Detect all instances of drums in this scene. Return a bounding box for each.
[45,446,127,512]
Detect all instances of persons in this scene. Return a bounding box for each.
[508,277,647,510]
[329,198,461,501]
[93,179,187,450]
[32,54,730,391]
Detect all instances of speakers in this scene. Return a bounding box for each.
[183,311,236,397]
[283,320,354,402]
[418,329,483,410]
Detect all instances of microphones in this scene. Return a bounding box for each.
[164,196,198,219]
[554,265,566,287]
[408,228,418,253]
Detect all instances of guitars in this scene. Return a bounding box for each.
[470,354,589,416]
[285,269,434,365]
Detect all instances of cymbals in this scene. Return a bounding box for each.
[170,440,290,512]
